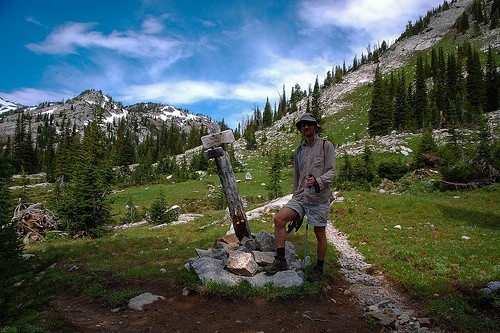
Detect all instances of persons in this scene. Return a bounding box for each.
[265,113,335,283]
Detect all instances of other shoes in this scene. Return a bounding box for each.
[264,257,288,273]
[308,270,324,282]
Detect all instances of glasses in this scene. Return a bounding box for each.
[298,122,314,127]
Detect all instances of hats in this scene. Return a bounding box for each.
[296,113,321,132]
